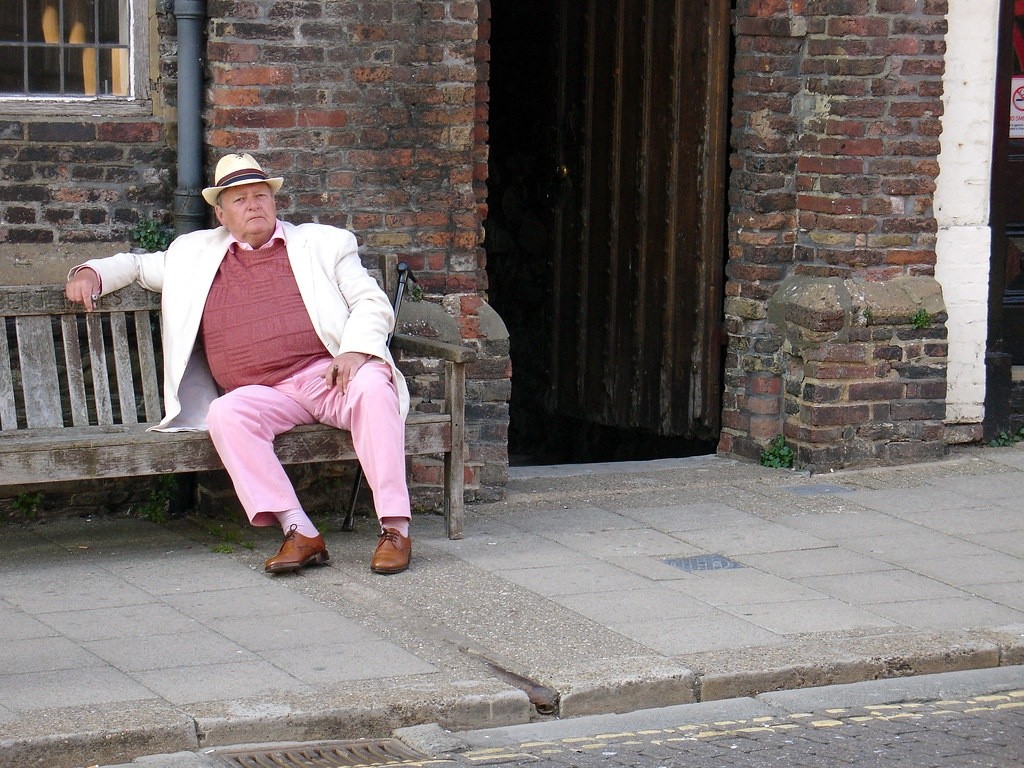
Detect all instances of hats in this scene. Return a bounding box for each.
[202,153,285,206]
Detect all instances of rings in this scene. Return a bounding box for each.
[91,295,98,305]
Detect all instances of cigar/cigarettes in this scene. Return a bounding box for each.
[333,364,339,387]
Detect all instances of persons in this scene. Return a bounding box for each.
[65,152,413,575]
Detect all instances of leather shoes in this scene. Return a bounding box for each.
[371,528,411,573]
[265,524,329,573]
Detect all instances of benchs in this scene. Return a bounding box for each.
[0,253,476,541]
